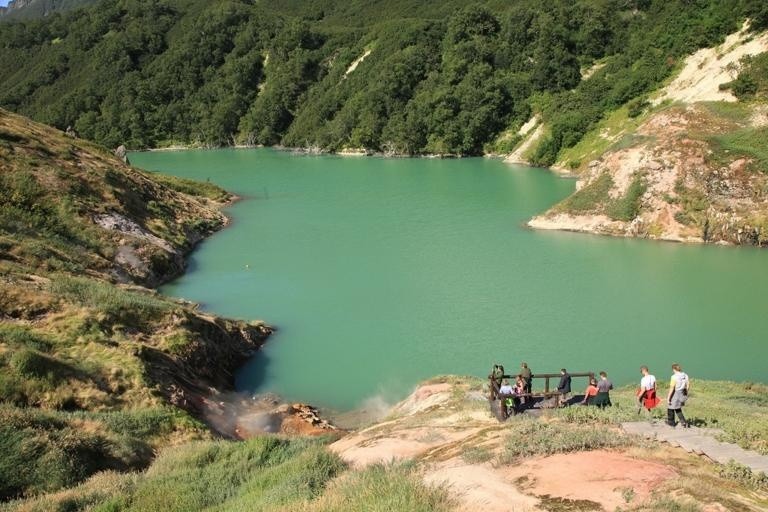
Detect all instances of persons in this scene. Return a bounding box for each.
[635,365,658,416]
[556,368,571,400]
[592,371,613,408]
[665,362,691,428]
[492,361,533,417]
[581,377,600,405]
